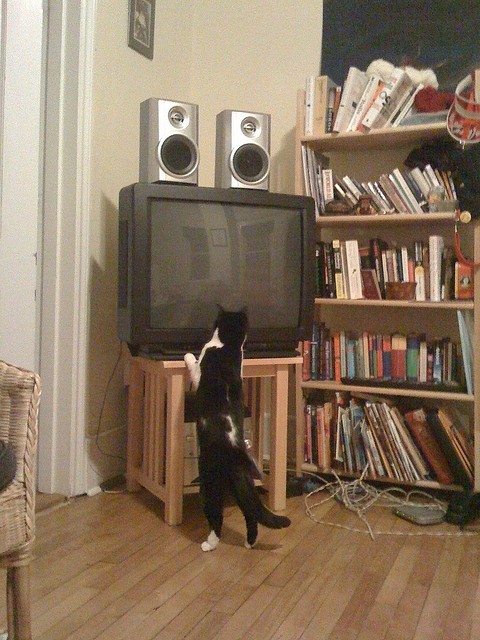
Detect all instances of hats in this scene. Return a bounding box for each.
[446,72,480,145]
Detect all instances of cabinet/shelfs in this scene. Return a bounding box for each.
[293,89,480,494]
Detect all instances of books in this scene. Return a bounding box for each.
[299,323,456,388]
[316,234,473,300]
[301,61,424,134]
[299,144,459,213]
[301,393,477,484]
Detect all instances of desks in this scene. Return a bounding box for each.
[129,357,303,525]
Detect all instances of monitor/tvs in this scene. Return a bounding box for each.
[116,181,317,362]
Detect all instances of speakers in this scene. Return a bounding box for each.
[138,97,200,186]
[214,108,272,192]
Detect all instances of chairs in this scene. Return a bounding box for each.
[0,361,42,639]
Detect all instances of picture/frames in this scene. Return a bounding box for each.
[128,1,154,61]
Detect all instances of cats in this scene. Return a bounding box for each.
[184,303,292,552]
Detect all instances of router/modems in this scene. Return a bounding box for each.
[391,505,447,526]
